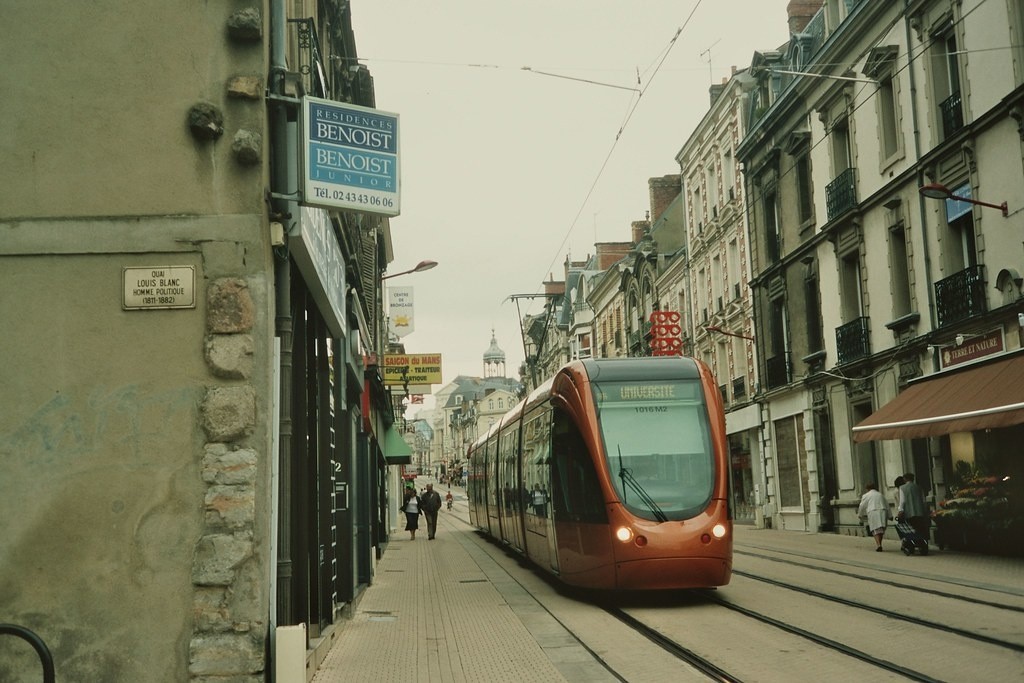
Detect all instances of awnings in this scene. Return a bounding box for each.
[851,353,1023,446]
[384,424,413,465]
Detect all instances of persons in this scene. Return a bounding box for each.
[445,490,453,509]
[857,483,894,552]
[894,472,929,553]
[503,482,549,518]
[403,487,423,540]
[421,483,441,540]
[403,485,428,502]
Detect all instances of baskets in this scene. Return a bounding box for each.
[448,499,452,503]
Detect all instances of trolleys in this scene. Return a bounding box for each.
[894,518,929,556]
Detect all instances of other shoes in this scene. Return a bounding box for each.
[428,536,435,540]
[876,546,882,551]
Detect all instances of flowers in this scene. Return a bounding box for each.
[926,460,1011,523]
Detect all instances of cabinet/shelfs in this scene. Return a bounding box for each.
[935,526,987,549]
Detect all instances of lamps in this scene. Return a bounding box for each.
[378,260,438,287]
[918,184,1008,217]
[1018,313,1024,328]
[927,344,953,355]
[706,326,755,343]
[403,397,424,406]
[956,333,987,345]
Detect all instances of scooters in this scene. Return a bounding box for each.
[447,497,454,510]
[466,355,734,598]
